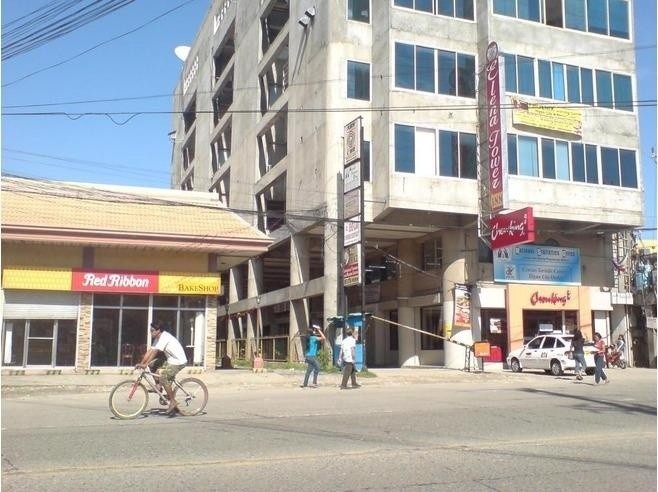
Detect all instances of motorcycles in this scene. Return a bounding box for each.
[603,344,628,370]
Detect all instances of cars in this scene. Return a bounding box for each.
[506,332,603,376]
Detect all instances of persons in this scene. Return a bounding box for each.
[568,330,588,380]
[335,328,361,389]
[616,334,626,362]
[299,325,327,389]
[590,331,612,386]
[135,319,188,419]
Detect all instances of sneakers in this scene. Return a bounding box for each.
[166,400,180,414]
[147,388,163,393]
[341,385,361,389]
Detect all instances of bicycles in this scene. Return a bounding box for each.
[108,363,212,419]
